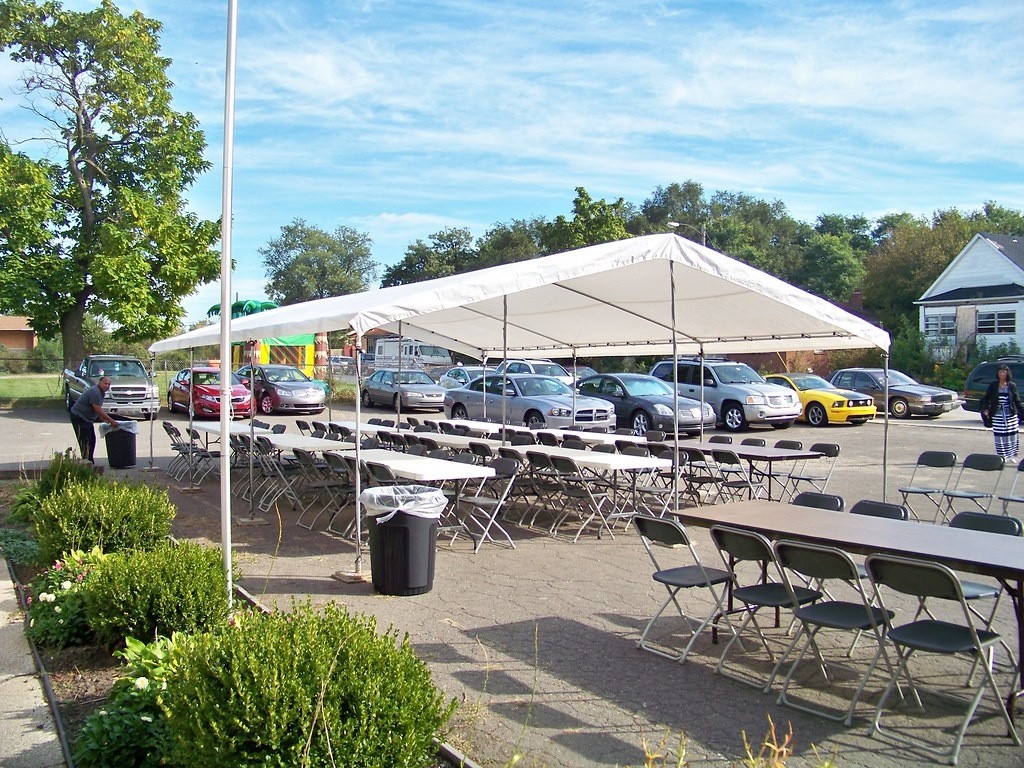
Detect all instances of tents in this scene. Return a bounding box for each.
[147,233,891,574]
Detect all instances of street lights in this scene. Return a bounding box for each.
[667,220,707,245]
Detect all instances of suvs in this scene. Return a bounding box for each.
[497,357,581,391]
[960,354,1024,421]
[646,355,803,432]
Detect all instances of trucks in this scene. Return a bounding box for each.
[375,335,452,369]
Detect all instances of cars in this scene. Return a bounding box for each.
[826,366,966,419]
[360,368,444,412]
[762,373,877,427]
[443,373,617,434]
[330,353,375,370]
[232,364,326,415]
[564,365,716,439]
[438,365,495,395]
[166,366,258,420]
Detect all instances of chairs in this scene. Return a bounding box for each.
[478,384,488,392]
[194,373,202,384]
[213,374,220,385]
[206,374,216,385]
[154,411,1024,768]
[582,382,596,390]
[523,380,537,395]
[268,370,279,381]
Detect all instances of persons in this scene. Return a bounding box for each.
[70,376,118,465]
[981,365,1024,464]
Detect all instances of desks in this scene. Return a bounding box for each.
[232,432,355,511]
[314,419,415,439]
[517,427,649,456]
[640,437,827,500]
[387,431,513,514]
[322,447,498,554]
[487,443,674,542]
[665,497,1024,740]
[186,420,274,482]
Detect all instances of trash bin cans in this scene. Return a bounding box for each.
[100,420,136,467]
[360,486,443,596]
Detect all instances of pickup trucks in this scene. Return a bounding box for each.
[62,354,161,420]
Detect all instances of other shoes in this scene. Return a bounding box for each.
[1002,459,1007,462]
[1009,459,1017,463]
